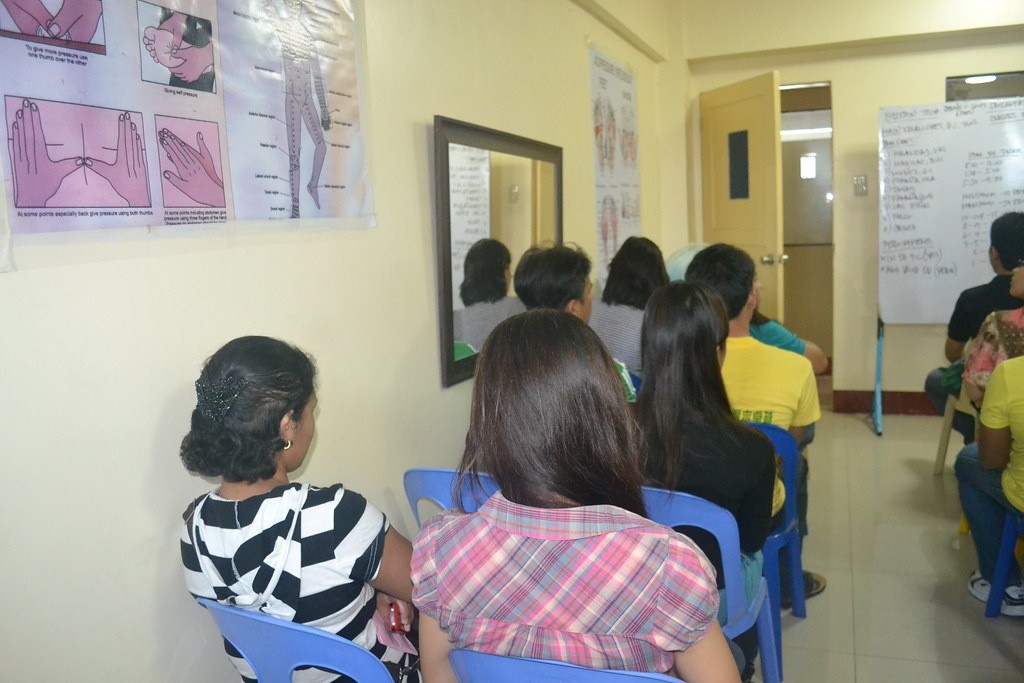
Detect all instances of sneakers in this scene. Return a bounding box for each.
[968,568,1024,616]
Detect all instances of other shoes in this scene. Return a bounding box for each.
[781,570,826,610]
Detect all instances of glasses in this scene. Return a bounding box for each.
[1014,259,1024,267]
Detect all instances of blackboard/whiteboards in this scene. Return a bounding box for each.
[877,95,1024,324]
[448,141,490,309]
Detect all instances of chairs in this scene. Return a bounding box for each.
[196,371,807,683]
[933,336,980,477]
[986,510,1024,617]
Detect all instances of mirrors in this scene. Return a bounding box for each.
[433,111,565,389]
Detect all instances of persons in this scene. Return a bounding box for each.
[684,245,827,609]
[749,311,827,453]
[955,355,1024,617]
[514,246,640,409]
[410,308,742,683]
[633,279,778,683]
[460,239,530,354]
[179,335,424,683]
[924,212,1024,446]
[962,252,1024,415]
[586,237,671,384]
